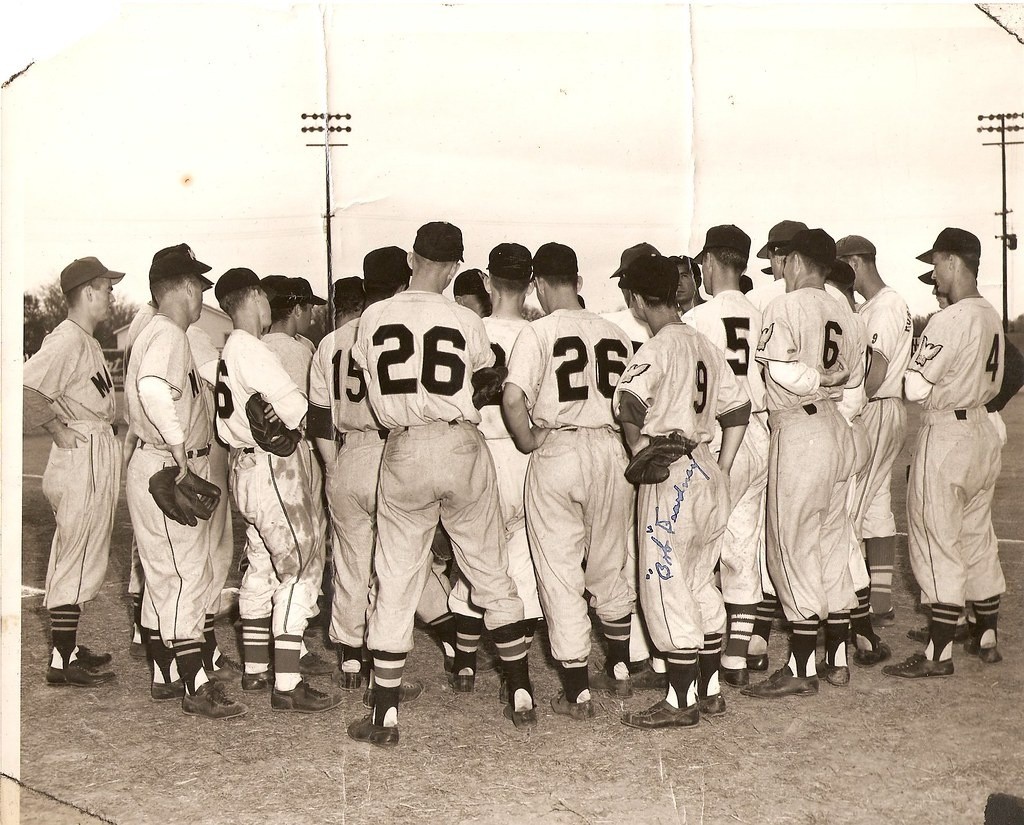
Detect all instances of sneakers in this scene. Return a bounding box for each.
[47,608,1003,749]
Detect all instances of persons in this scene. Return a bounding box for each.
[348,222,537,746]
[23,219,1024,726]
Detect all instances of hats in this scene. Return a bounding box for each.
[669,254,701,280]
[334,276,365,299]
[529,242,578,282]
[364,246,413,282]
[918,270,936,285]
[835,235,876,257]
[758,219,809,259]
[413,221,464,263]
[260,275,288,292]
[825,260,856,284]
[271,277,328,306]
[609,242,661,279]
[60,257,125,292]
[617,254,679,295]
[775,227,836,258]
[692,224,751,264]
[453,269,489,297]
[487,243,533,271]
[214,268,262,301]
[916,227,981,265]
[149,243,215,292]
[739,275,753,294]
[761,267,773,275]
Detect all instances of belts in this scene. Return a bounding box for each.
[139,402,976,459]
